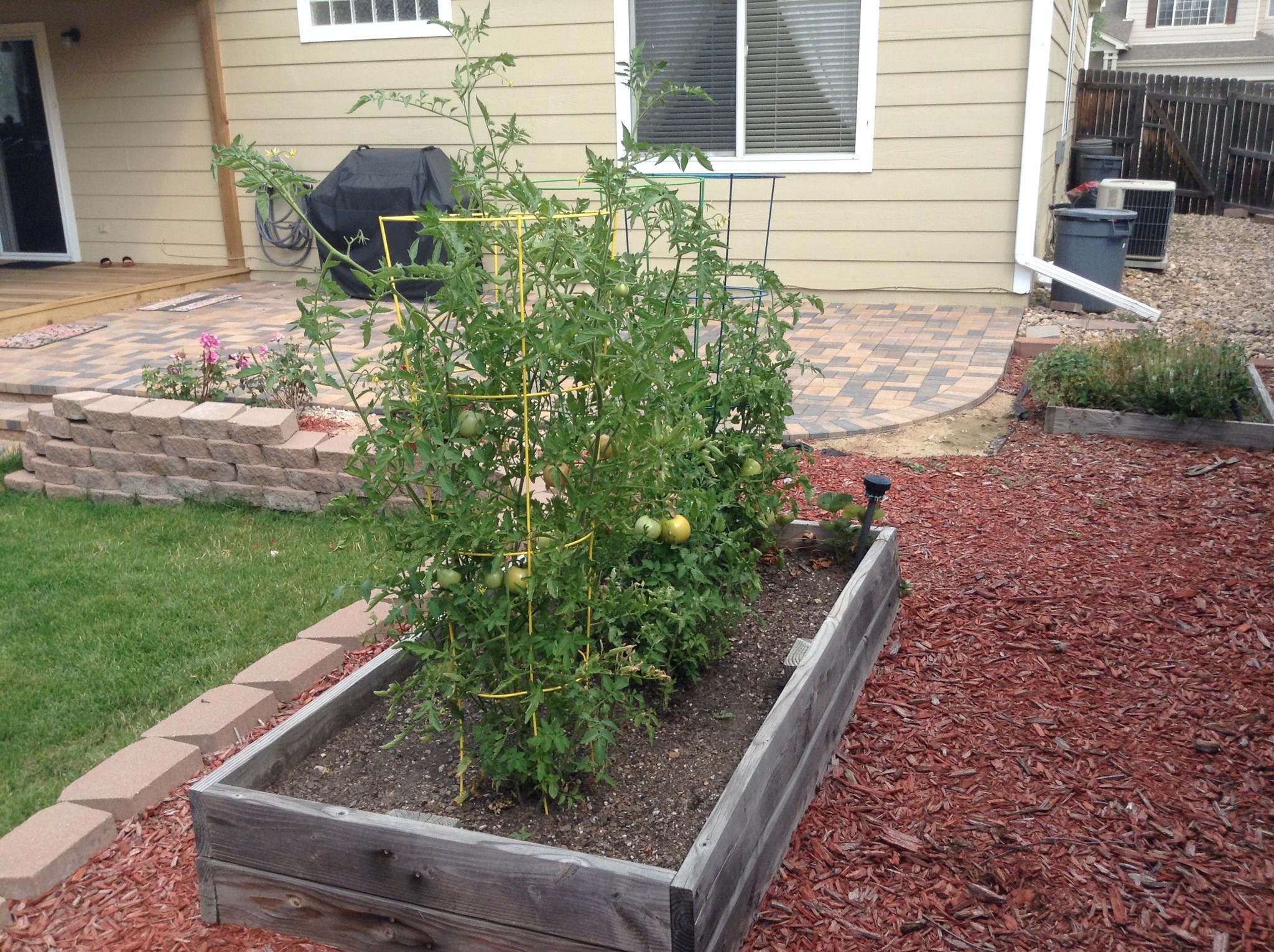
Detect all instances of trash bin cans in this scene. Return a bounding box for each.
[1081,153,1124,183]
[1074,138,1114,185]
[1051,207,1138,315]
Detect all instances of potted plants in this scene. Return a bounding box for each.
[163,0,895,952]
[1015,327,1274,448]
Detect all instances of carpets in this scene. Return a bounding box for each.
[0,323,108,349]
[0,261,76,270]
[136,291,242,312]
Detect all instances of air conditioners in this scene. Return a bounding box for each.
[1096,178,1176,262]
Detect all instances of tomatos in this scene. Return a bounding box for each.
[436,409,764,594]
[614,283,630,297]
